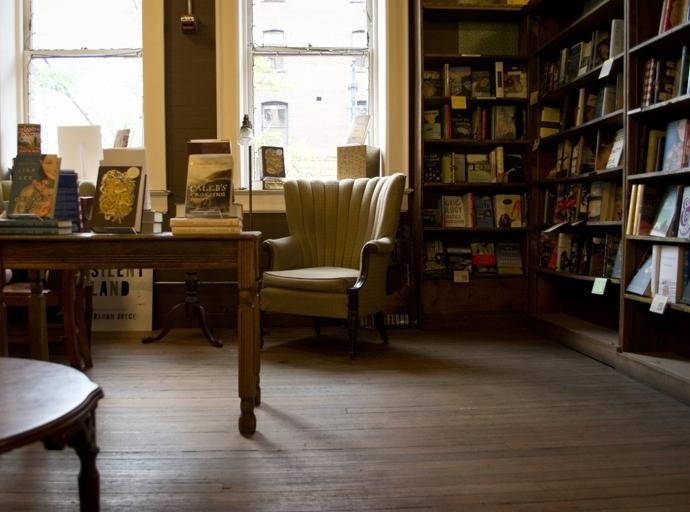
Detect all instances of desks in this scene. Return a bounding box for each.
[1,230,264,438]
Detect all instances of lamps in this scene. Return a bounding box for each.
[237,114,254,231]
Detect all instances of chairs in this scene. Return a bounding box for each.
[0,268,96,370]
[256,173,407,361]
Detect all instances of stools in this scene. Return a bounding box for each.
[0,358,105,510]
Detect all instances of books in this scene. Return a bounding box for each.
[548,129,623,177]
[544,182,622,224]
[538,232,622,277]
[426,62,527,97]
[426,146,521,183]
[0,123,162,235]
[626,245,688,303]
[643,46,688,106]
[168,139,244,236]
[540,74,622,138]
[253,145,286,190]
[426,241,523,275]
[427,104,525,140]
[542,20,624,93]
[658,1,689,35]
[426,192,528,228]
[626,185,688,238]
[645,119,689,172]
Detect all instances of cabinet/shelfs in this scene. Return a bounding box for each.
[532,1,689,400]
[407,0,536,330]
